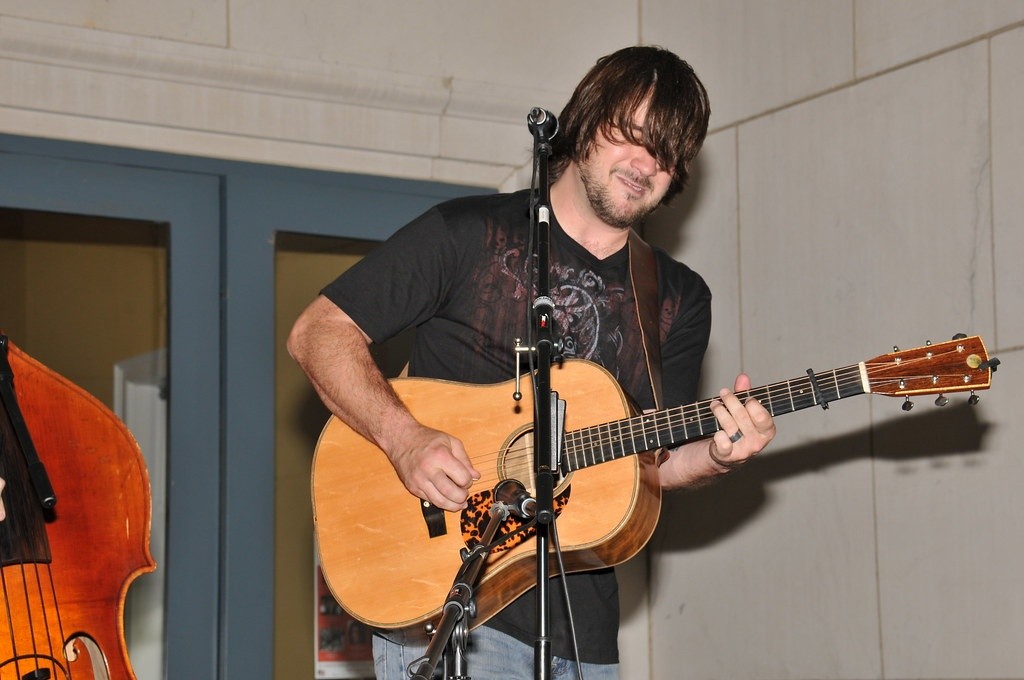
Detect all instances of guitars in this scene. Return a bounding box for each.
[312,333,999,646]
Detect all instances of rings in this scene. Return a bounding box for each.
[729,429,743,443]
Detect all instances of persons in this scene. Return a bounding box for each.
[286,44,777,680]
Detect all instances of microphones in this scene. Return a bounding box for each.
[527,105,561,141]
[492,480,537,518]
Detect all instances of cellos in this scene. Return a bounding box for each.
[0,333,159,680]
